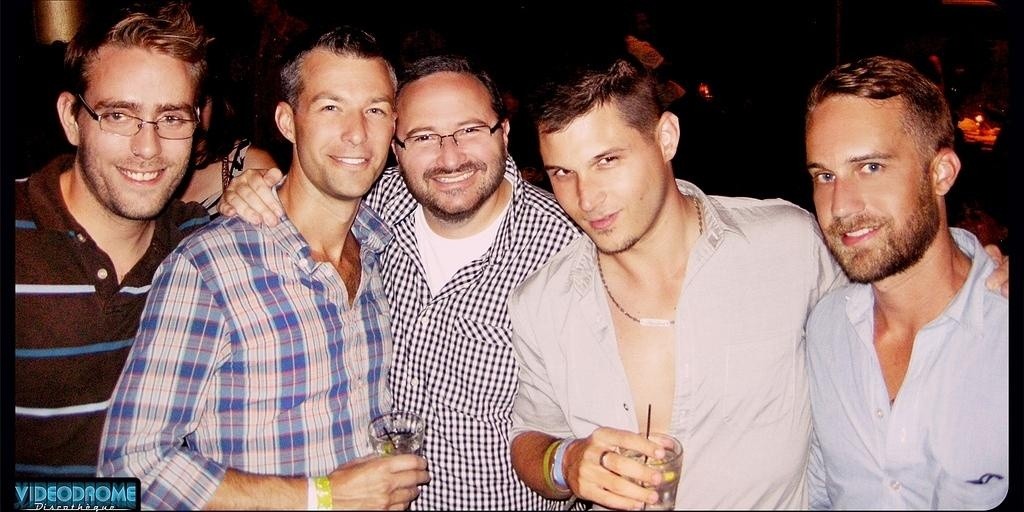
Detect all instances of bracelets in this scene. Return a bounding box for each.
[308,478,317,510]
[542,438,574,498]
[314,476,332,510]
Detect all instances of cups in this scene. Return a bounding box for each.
[616,432,681,511]
[367,413,423,458]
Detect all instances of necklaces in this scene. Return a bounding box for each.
[597,194,702,328]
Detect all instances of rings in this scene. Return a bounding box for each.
[599,450,618,471]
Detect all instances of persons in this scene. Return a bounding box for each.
[173,61,279,222]
[214,54,587,511]
[95,24,433,511]
[806,55,1009,511]
[507,55,1009,511]
[15,3,211,477]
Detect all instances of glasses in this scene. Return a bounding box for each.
[78,94,201,140]
[393,121,501,154]
